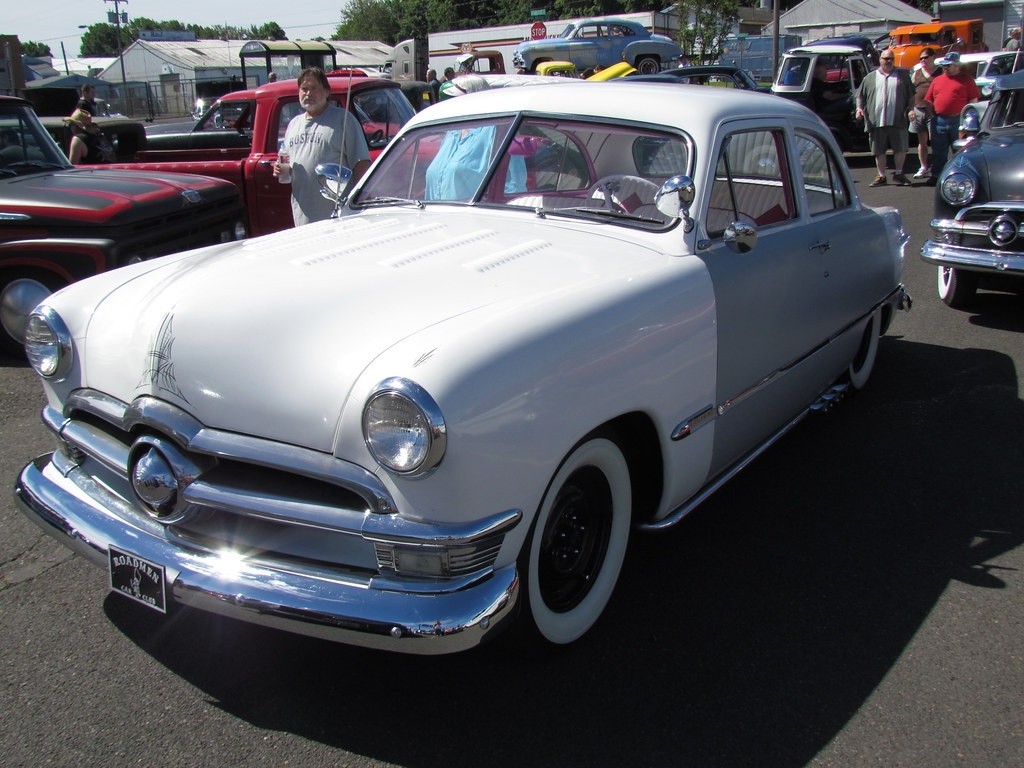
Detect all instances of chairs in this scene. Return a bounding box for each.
[630,204,759,233]
[503,193,625,213]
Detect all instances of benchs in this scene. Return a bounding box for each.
[593,176,836,224]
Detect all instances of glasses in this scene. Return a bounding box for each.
[881,57,894,61]
[920,56,929,60]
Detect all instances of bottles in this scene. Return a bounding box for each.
[278,144,290,183]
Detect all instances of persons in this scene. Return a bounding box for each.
[62,109,115,166]
[268,72,276,83]
[76,84,97,115]
[581,64,604,79]
[273,67,372,227]
[923,52,981,186]
[1002,28,1022,51]
[438,67,457,100]
[908,48,943,178]
[425,75,528,201]
[426,69,441,103]
[855,49,916,187]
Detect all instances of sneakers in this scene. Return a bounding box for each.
[893,172,911,185]
[913,168,928,179]
[869,176,887,186]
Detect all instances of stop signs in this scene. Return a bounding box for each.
[530,21,547,40]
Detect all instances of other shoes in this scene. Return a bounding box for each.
[927,176,937,185]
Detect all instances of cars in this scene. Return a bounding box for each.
[511,15,685,76]
[1,21,1024,208]
[11,81,916,670]
[0,96,248,360]
[919,68,1024,311]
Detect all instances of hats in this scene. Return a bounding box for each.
[63,108,98,135]
[443,83,467,97]
[939,52,961,65]
